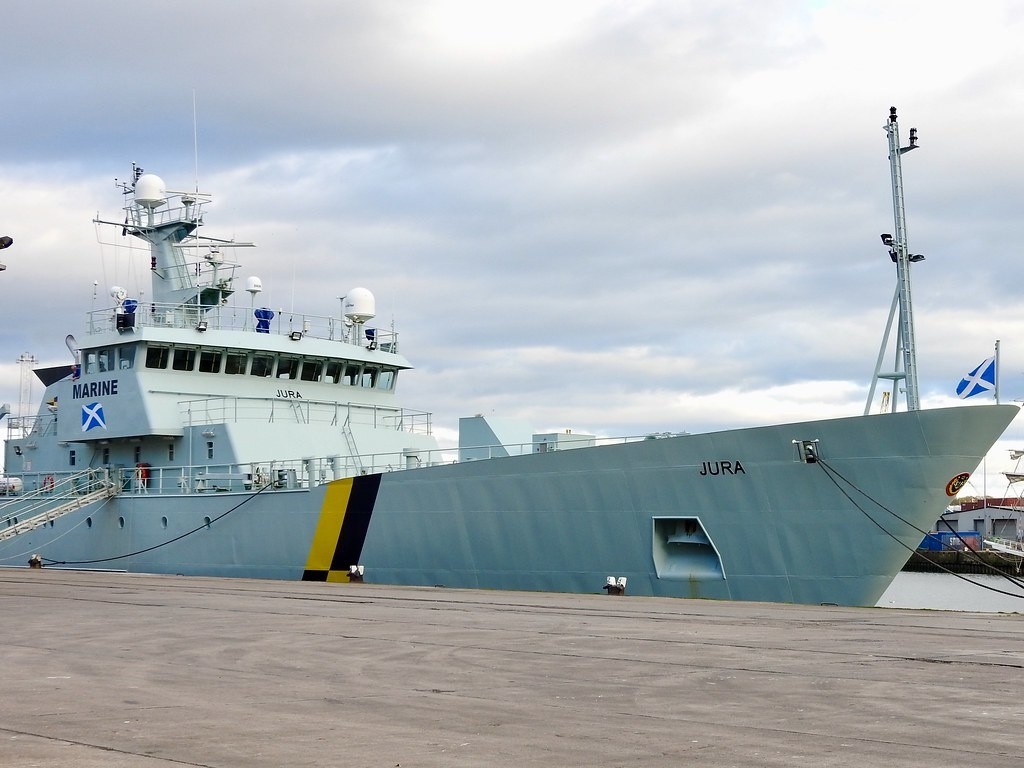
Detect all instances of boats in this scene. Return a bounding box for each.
[0,88,1019,610]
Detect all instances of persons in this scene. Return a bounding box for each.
[69,366,80,381]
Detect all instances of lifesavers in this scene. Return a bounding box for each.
[136,464,148,481]
[43,477,54,492]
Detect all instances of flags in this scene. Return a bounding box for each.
[956,355,997,399]
